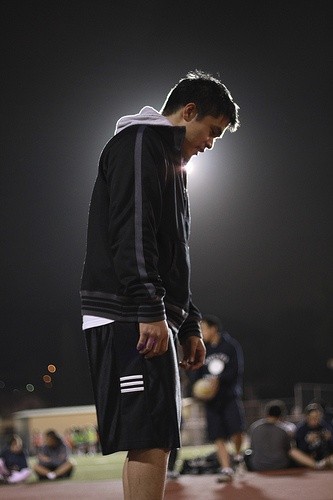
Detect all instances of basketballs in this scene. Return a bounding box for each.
[193,379,215,400]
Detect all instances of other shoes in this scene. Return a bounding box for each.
[9,469,29,483]
[217,460,248,483]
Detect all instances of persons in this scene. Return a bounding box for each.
[34,430,73,481]
[32,424,104,454]
[0,433,31,482]
[80,69,239,500]
[244,402,295,473]
[191,315,244,483]
[286,402,333,471]
